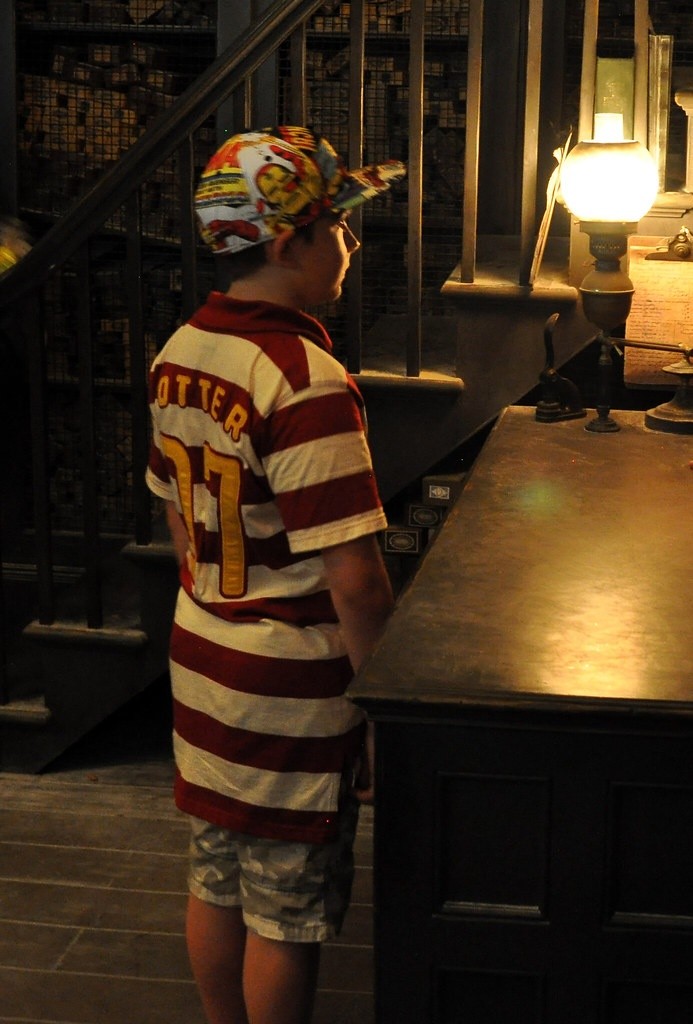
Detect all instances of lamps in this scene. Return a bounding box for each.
[559,113,659,432]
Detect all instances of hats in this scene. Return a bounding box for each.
[193,125,406,256]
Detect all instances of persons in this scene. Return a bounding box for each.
[141,122,412,1024]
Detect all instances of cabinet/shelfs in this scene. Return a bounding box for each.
[351,402,693,1023]
